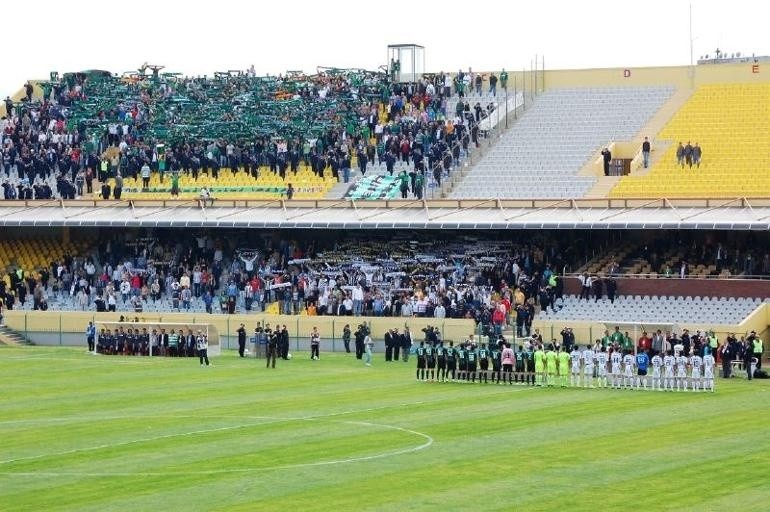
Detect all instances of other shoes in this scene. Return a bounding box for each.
[93,350,211,366]
[536,376,714,392]
[265,353,289,368]
[240,354,244,357]
[416,377,535,387]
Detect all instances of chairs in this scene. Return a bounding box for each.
[0,73,769,203]
[0,227,770,326]
